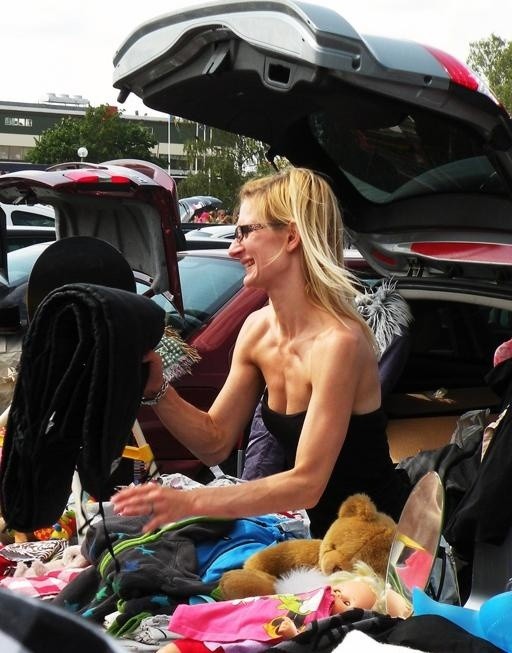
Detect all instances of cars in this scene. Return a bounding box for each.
[111,0,512,469]
[184,224,363,259]
[1,203,57,329]
[1,158,369,469]
[179,194,223,223]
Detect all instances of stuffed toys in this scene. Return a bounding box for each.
[14,542,90,580]
[34,508,78,541]
[223,491,400,601]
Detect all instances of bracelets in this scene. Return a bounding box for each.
[141,379,168,404]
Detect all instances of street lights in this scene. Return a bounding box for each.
[78,146,89,162]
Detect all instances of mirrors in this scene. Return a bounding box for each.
[385,471,446,619]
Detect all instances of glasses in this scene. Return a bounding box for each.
[233,221,290,243]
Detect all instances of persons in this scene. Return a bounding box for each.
[111,169,411,539]
[155,558,387,653]
[193,208,232,224]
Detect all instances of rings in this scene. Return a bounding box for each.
[148,502,153,515]
